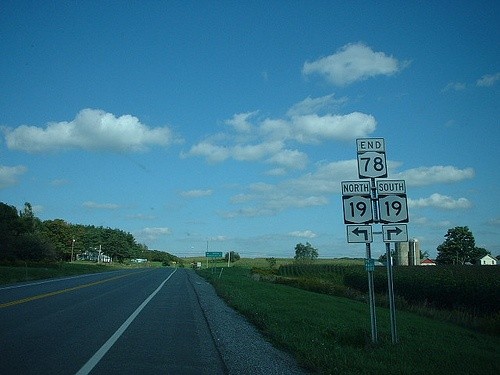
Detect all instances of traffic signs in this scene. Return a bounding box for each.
[342,137,410,243]
